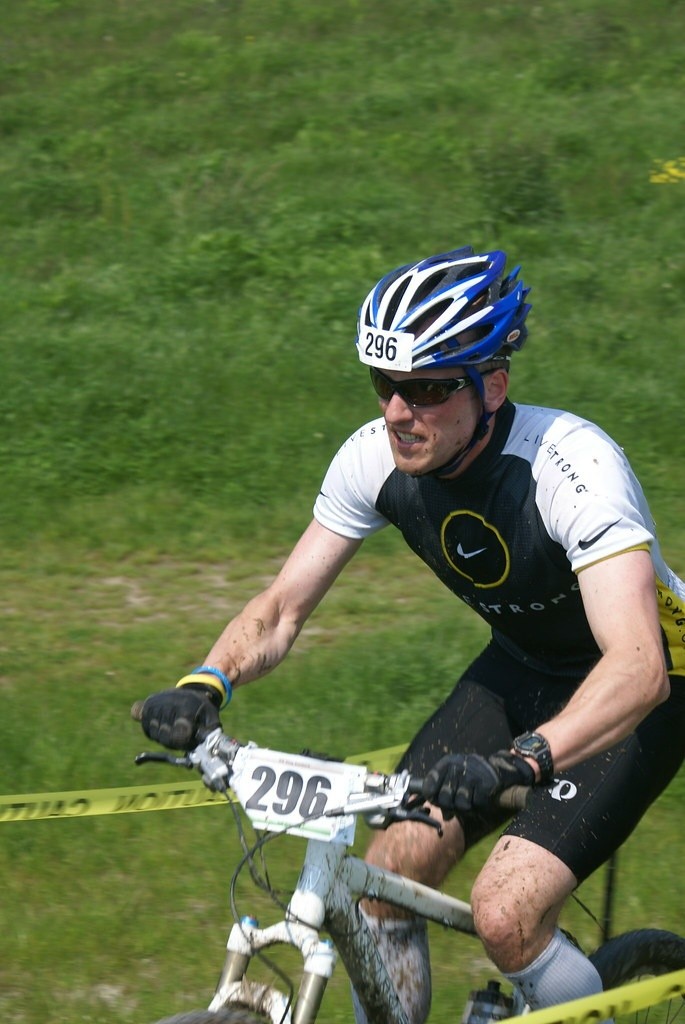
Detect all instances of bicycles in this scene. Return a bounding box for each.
[128,697,685,1023]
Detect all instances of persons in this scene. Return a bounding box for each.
[141,246,685,1024]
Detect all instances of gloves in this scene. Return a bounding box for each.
[421,749,535,821]
[141,682,223,750]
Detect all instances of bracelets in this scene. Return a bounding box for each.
[175,666,231,710]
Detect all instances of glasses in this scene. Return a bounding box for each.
[370,366,508,408]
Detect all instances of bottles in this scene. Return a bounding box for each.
[466,980,513,1024]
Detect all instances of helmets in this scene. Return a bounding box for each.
[356,245,532,373]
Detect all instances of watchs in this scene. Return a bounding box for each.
[514,731,554,783]
